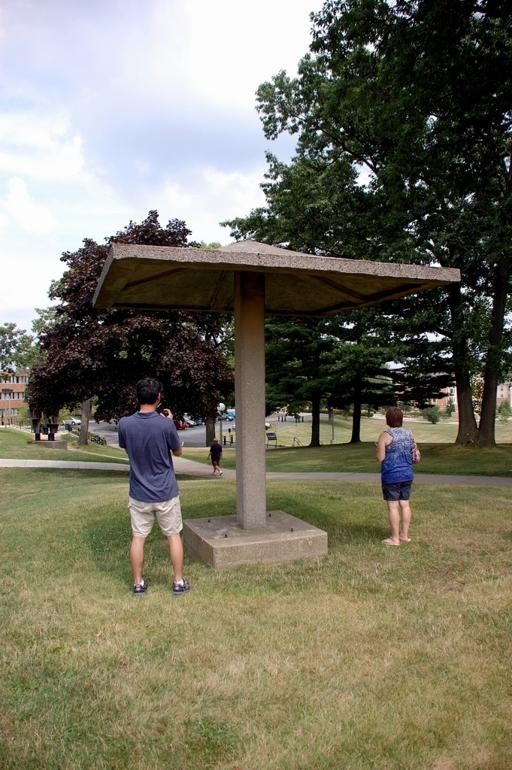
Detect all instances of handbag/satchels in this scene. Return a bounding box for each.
[412,449,421,464]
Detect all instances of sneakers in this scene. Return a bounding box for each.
[173,578,191,595]
[134,580,147,596]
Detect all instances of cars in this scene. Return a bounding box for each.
[217,409,235,421]
[60,416,81,426]
[173,413,207,430]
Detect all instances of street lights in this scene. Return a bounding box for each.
[216,402,226,446]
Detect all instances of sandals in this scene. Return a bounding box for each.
[382,536,411,545]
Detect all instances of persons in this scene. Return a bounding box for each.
[376,408,421,546]
[207,440,223,476]
[118,377,190,593]
